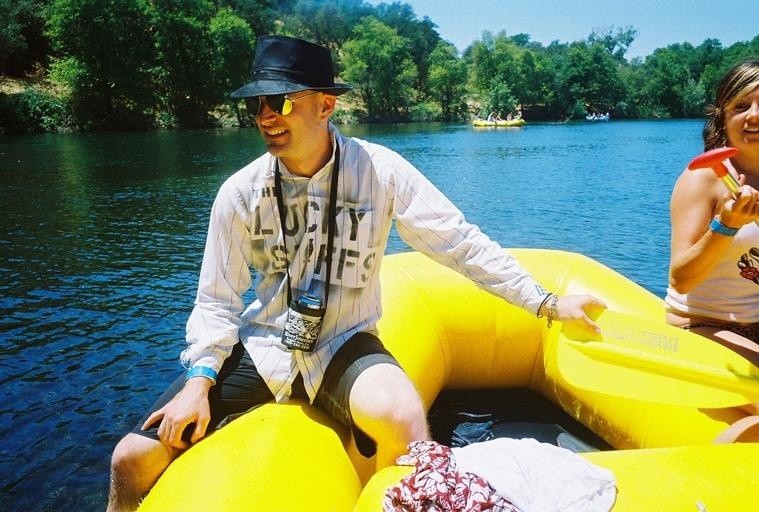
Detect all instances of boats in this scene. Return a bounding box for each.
[472,115,609,126]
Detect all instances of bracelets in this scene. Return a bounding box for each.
[544,293,562,331]
[183,366,220,383]
[710,213,741,238]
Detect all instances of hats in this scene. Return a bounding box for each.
[229,34,354,100]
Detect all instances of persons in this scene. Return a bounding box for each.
[664,57,759,370]
[597,112,601,118]
[487,110,522,122]
[103,36,609,512]
[474,111,482,121]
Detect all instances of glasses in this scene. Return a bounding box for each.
[245,91,323,117]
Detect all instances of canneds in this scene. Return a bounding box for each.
[298,295,324,310]
